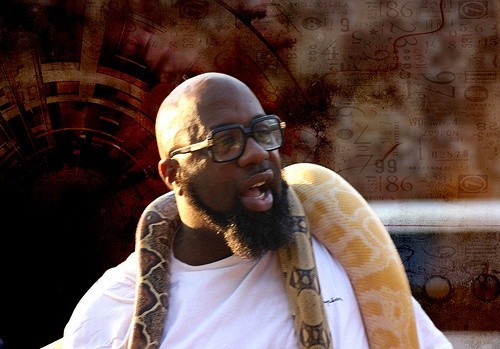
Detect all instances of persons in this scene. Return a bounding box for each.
[37,72,455,348]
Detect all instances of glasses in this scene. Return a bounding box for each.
[170,114,286,163]
